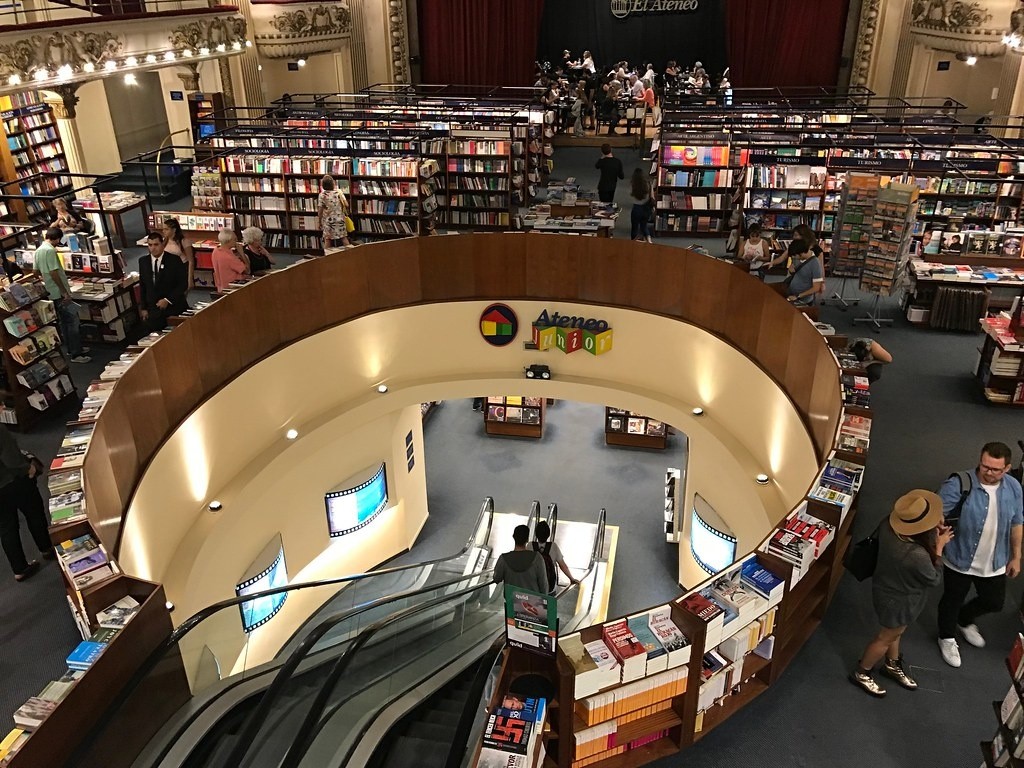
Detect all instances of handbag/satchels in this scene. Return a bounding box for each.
[784,274,794,288]
[345,215,356,232]
[20,448,45,476]
[841,535,877,584]
[646,198,657,213]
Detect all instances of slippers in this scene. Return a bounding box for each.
[14,561,39,583]
[41,550,50,559]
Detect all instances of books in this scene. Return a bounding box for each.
[475,106,1024,768]
[0,89,556,768]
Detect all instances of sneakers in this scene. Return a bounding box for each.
[956,621,986,648]
[67,346,90,357]
[71,356,92,364]
[937,637,962,667]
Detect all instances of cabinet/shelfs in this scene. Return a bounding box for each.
[1,83,1024,768]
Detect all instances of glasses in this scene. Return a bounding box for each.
[981,464,1006,475]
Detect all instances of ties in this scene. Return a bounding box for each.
[154,258,158,284]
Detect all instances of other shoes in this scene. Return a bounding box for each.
[846,660,887,698]
[880,654,917,690]
[608,129,619,137]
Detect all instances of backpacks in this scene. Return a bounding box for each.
[178,238,198,271]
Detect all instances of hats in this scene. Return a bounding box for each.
[564,50,571,53]
[696,62,702,68]
[889,488,943,536]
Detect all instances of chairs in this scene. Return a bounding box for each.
[538,61,724,137]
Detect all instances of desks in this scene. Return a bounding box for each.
[552,103,571,135]
[619,97,639,136]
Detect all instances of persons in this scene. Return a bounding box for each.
[502,694,527,713]
[241,226,277,277]
[48,196,91,234]
[212,230,252,294]
[787,238,825,307]
[39,342,49,352]
[32,227,92,364]
[742,223,770,263]
[54,334,61,345]
[847,487,955,697]
[934,442,1024,668]
[0,401,56,581]
[527,520,579,593]
[628,166,658,244]
[844,337,893,386]
[159,217,195,292]
[318,175,350,249]
[762,223,824,271]
[138,232,191,333]
[595,143,624,210]
[949,235,963,250]
[923,230,934,248]
[532,47,712,136]
[492,525,550,595]
